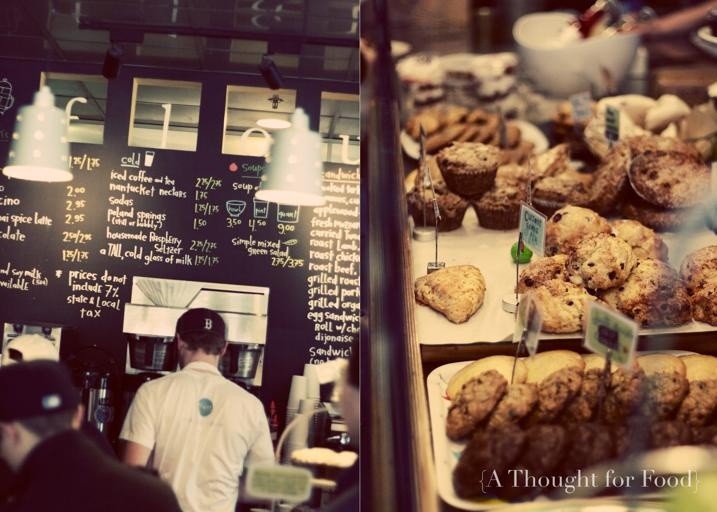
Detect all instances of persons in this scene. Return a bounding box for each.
[118,308,278,512]
[0,332,118,459]
[622,1,717,37]
[330,336,360,443]
[0,359,181,512]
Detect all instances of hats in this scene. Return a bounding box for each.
[175,308,224,341]
[2,362,77,420]
[3,336,61,365]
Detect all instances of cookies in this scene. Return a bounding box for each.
[407,102,524,149]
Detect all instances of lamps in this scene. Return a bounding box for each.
[254,107,325,208]
[102,44,124,80]
[2,85,74,184]
[258,54,285,90]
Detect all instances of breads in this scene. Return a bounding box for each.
[515,205,717,333]
[414,264,486,323]
[407,137,712,232]
[445,349,716,502]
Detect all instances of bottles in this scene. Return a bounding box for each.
[95,377,113,435]
[80,371,98,425]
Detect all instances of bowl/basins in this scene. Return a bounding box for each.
[511,11,636,92]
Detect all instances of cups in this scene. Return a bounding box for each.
[285,374,320,465]
